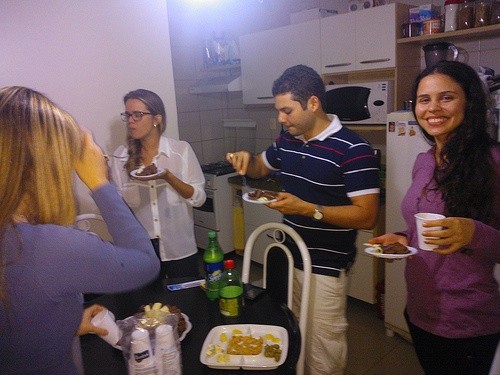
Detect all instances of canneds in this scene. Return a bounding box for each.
[422,18,441,35]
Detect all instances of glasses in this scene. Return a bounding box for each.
[121,111,156,122]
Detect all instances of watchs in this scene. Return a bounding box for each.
[313,204,323,220]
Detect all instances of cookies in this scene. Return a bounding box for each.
[225,334,264,355]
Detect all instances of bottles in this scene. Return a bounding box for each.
[203,231,224,300]
[219,260,245,325]
[444,0,492,32]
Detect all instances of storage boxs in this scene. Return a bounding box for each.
[290,8,338,25]
[409,4,439,24]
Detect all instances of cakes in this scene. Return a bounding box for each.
[372,242,411,254]
[247,188,278,200]
[135,162,158,175]
[135,302,187,339]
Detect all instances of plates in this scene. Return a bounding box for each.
[242,191,278,204]
[364,246,417,258]
[108,154,128,159]
[199,323,289,370]
[130,167,164,181]
[113,313,192,352]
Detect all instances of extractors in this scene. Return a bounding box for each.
[190,71,242,94]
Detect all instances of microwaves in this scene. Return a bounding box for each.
[324,79,395,124]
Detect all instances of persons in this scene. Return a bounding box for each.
[370,59,500,375]
[105,89,207,275]
[0,85,158,374]
[227,63,380,375]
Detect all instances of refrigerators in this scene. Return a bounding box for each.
[385,112,434,345]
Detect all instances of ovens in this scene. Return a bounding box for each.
[193,190,235,254]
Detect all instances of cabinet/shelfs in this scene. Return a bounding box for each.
[242,186,384,305]
[190,3,500,131]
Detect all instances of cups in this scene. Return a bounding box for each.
[90,310,123,346]
[128,324,181,375]
[415,213,446,251]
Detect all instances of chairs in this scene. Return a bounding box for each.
[242,223,312,375]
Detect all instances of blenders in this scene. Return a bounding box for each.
[423,41,459,68]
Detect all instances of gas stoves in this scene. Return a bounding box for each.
[200,159,239,190]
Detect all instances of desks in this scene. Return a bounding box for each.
[80,277,303,375]
